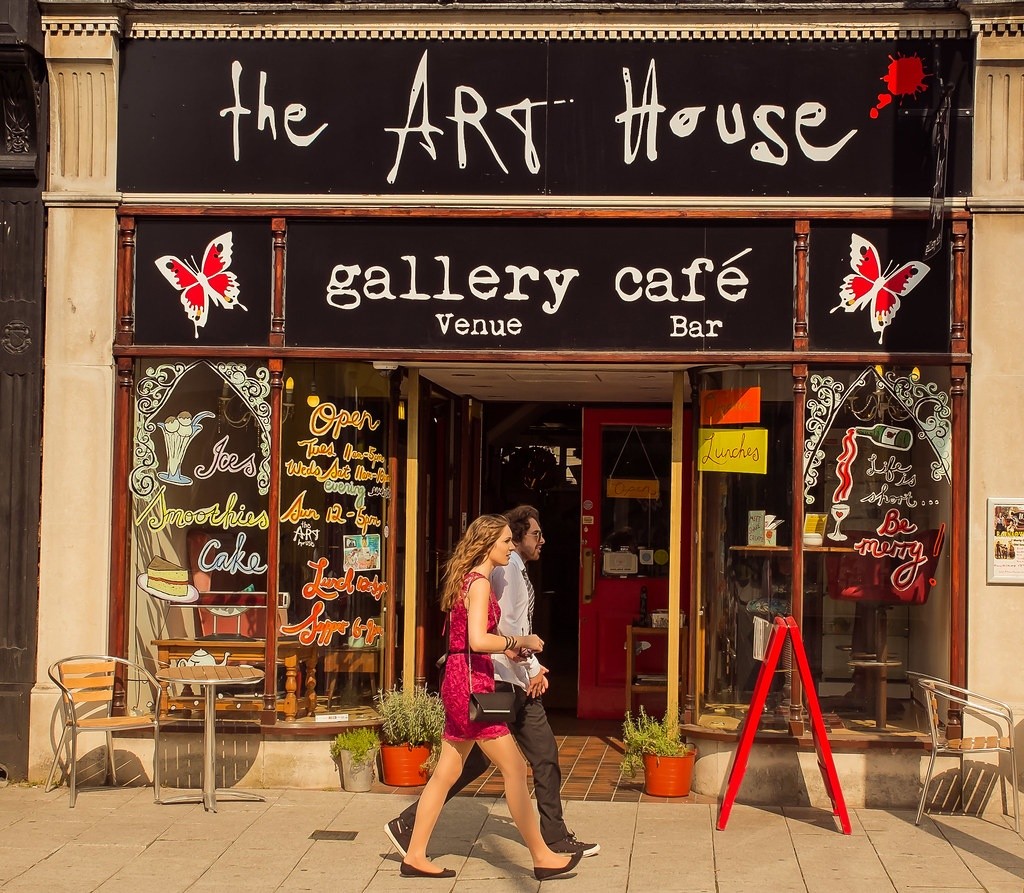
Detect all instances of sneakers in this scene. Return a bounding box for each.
[546,829,601,858]
[383,816,431,862]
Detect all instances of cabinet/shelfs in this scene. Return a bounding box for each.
[626,625,688,720]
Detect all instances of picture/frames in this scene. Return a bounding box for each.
[985,497,1023,585]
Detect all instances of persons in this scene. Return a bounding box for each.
[384,506,600,863]
[401,517,583,880]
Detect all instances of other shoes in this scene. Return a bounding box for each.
[401,861,456,878]
[534,850,584,880]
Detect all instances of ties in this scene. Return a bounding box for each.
[522,571,535,635]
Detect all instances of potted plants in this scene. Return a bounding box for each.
[373,683,446,786]
[329,726,381,793]
[619,703,697,798]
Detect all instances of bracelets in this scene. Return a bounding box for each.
[504,636,517,651]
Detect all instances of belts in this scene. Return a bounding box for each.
[450,651,488,654]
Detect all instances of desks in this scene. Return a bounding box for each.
[324,648,380,709]
[150,641,319,723]
[727,545,859,731]
[153,665,267,814]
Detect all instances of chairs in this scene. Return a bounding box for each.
[46,655,163,808]
[915,680,1021,835]
[186,528,288,637]
[826,525,944,733]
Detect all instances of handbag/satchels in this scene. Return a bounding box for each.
[469,692,517,722]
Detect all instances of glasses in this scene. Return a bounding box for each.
[523,530,544,542]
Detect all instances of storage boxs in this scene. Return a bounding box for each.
[652,609,684,628]
